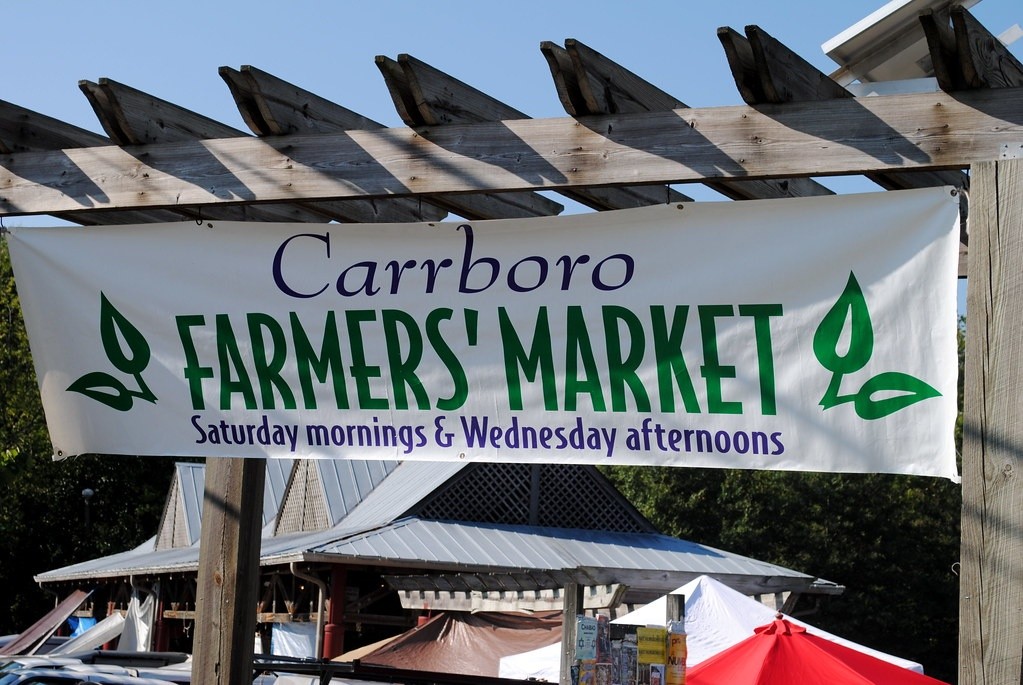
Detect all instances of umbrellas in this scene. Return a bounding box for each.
[686,612,952,685]
[498,574,925,683]
